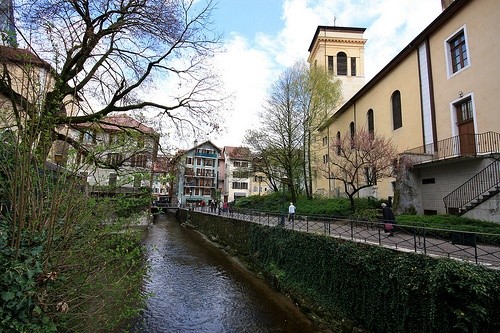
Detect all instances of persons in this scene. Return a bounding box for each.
[287,202,296,222]
[200,199,228,215]
[382,203,396,236]
